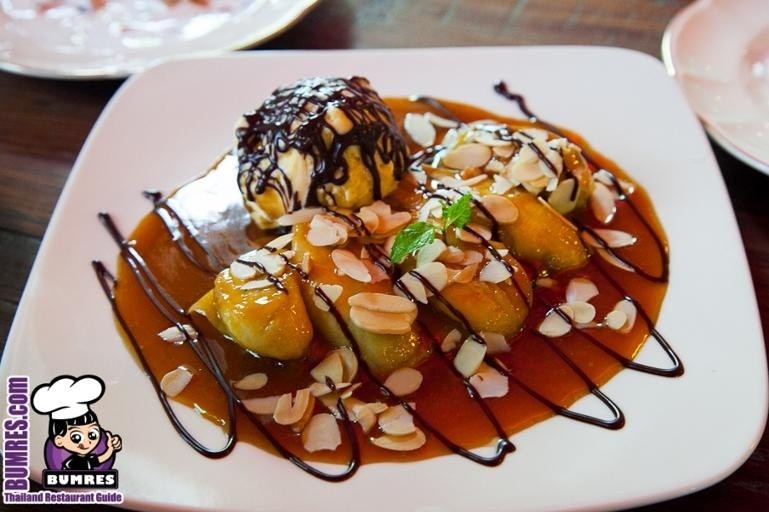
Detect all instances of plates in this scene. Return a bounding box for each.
[1,48,762,508]
[2,3,318,80]
[662,0,764,170]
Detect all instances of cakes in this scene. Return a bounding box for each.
[237,77,410,231]
